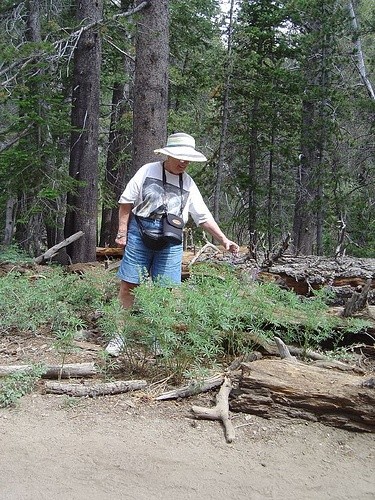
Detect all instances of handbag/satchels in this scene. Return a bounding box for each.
[162,212,184,244]
[135,216,170,251]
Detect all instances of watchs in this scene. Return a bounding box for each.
[116,232,127,239]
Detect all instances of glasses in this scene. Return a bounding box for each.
[172,157,190,165]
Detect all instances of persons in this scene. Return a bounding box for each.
[105,133,240,358]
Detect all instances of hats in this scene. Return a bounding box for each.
[154,132,208,163]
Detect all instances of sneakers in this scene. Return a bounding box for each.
[149,342,165,358]
[106,335,127,357]
